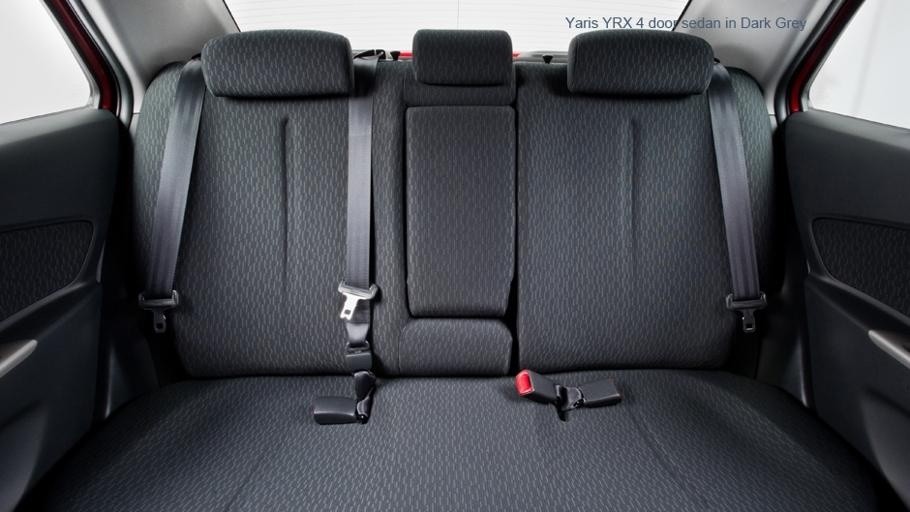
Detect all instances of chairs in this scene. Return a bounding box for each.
[20,30,899,511]
